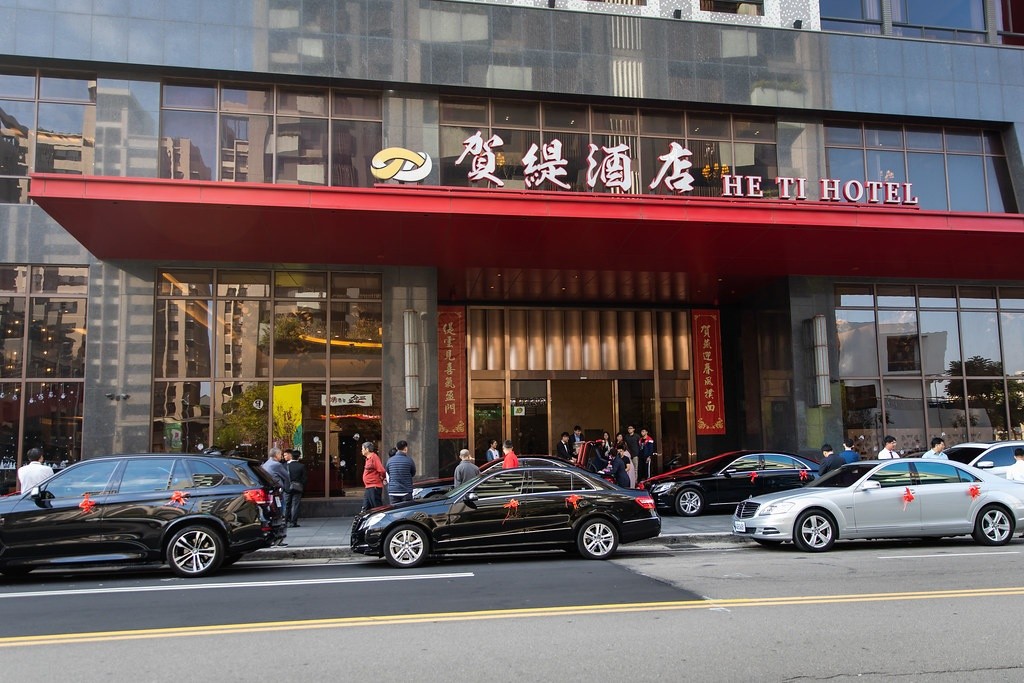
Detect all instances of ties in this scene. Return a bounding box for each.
[889,451,893,459]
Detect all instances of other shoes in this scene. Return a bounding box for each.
[290,524,301,528]
[270,541,288,548]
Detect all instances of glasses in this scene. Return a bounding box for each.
[627,427,634,430]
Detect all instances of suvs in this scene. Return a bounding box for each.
[0,452,287,582]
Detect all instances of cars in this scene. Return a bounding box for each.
[350,465,663,569]
[941,440,1024,480]
[730,457,1024,554]
[638,449,821,518]
[411,454,617,500]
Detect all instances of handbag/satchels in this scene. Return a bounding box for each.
[289,481,303,494]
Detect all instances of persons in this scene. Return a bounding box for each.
[362,442,387,511]
[263,448,291,548]
[1007,449,1024,481]
[878,436,900,459]
[18,449,54,494]
[839,439,860,463]
[454,449,480,488]
[487,440,500,462]
[557,432,577,463]
[568,427,585,458]
[603,425,654,488]
[922,438,948,459]
[502,440,518,468]
[283,448,307,527]
[386,441,417,505]
[818,444,846,476]
